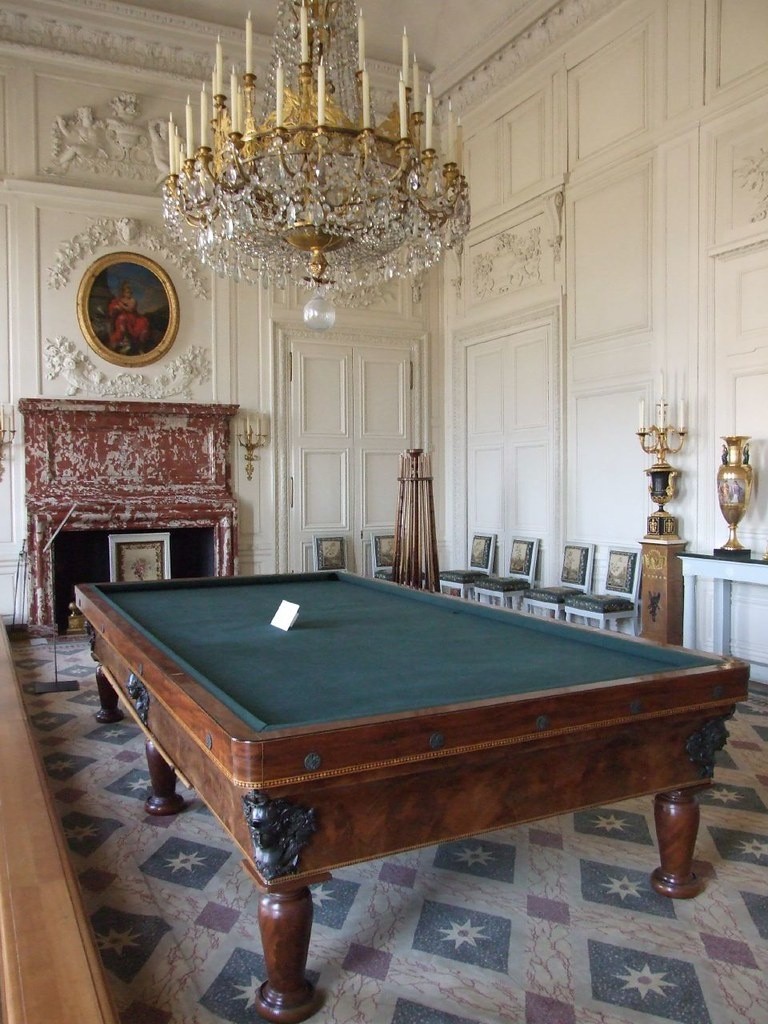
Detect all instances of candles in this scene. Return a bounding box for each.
[678,397,686,430]
[659,399,665,428]
[637,399,647,429]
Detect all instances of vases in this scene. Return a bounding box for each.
[713,436,756,559]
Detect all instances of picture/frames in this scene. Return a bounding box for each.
[80,251,183,368]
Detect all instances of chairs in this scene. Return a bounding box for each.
[523,542,597,619]
[473,536,540,610]
[108,532,171,582]
[438,531,497,600]
[311,532,349,573]
[372,531,398,580]
[565,546,642,636]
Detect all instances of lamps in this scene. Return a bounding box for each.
[158,1,472,334]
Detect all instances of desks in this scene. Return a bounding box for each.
[73,565,751,1023]
